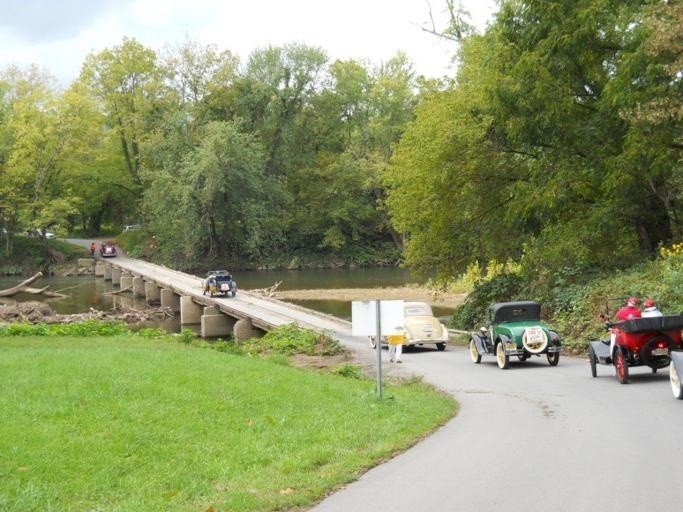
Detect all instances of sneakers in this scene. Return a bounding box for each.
[390,359,401,363]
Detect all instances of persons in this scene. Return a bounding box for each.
[614,297,640,320]
[90,242,96,256]
[639,298,662,317]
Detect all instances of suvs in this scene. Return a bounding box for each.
[584,293,682,386]
[468,300,561,371]
[199,268,238,299]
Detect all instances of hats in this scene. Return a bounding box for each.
[628,298,638,304]
[645,300,654,307]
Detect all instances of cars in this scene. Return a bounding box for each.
[119,225,141,234]
[362,300,448,353]
[665,344,682,400]
[98,241,117,257]
[23,228,57,240]
